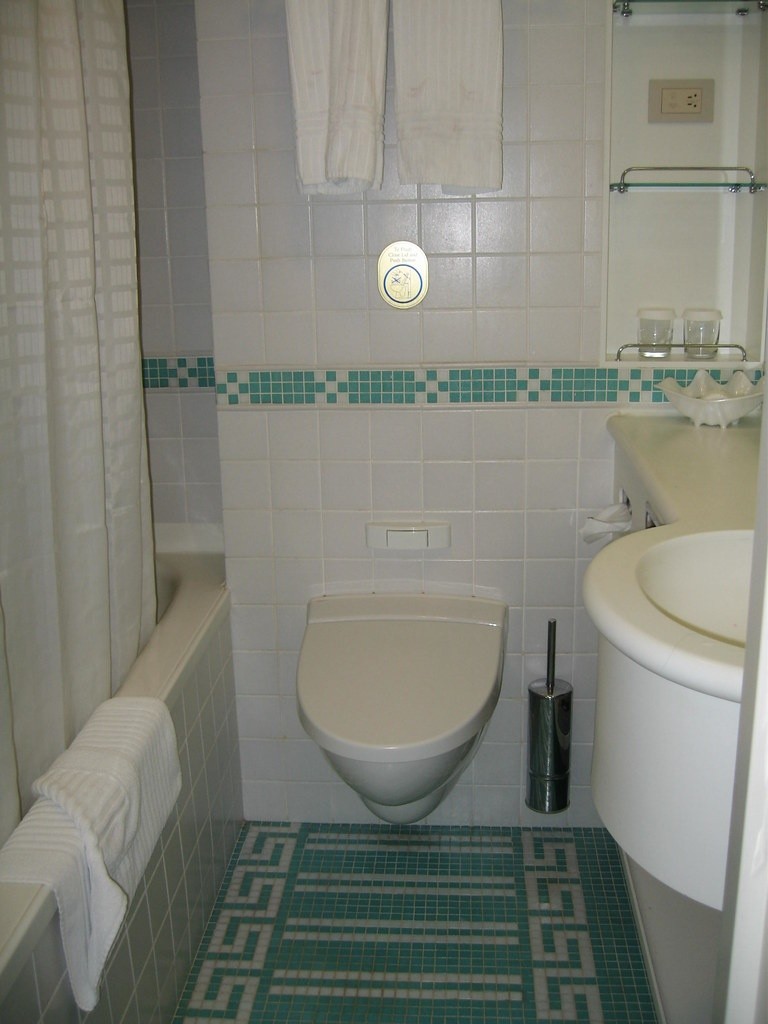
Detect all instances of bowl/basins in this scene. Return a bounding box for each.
[654,369,765,428]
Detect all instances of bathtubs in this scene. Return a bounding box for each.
[2,554,245,1022]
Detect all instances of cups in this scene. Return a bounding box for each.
[638,309,675,358]
[683,311,721,359]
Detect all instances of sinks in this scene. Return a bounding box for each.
[582,521,757,916]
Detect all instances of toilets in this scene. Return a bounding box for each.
[297,582,501,820]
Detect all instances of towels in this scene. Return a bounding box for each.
[388,0,501,193]
[1,698,184,1014]
[280,0,387,195]
